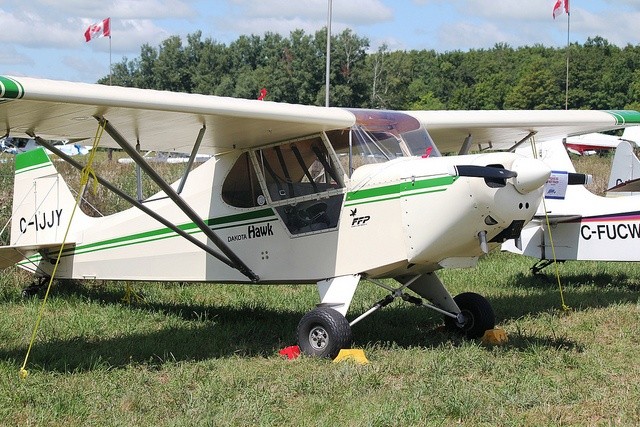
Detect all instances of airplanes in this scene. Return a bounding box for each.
[0,75,640,362]
[501,125,640,277]
